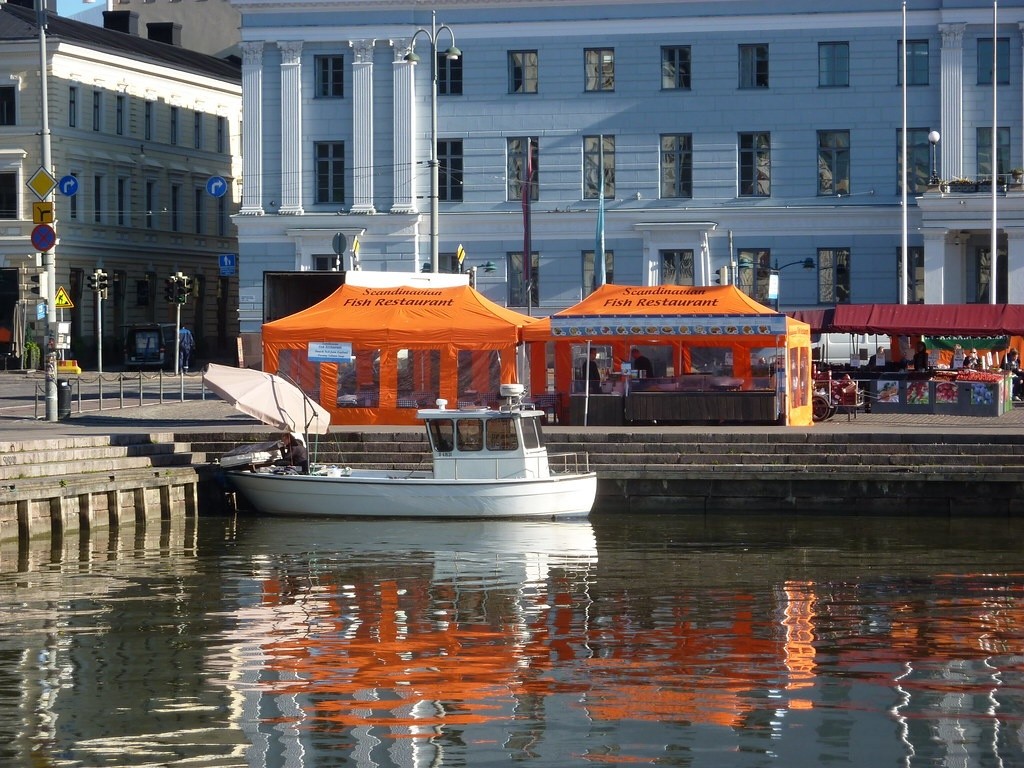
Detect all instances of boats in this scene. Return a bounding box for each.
[221,514,603,590]
[217,385,601,523]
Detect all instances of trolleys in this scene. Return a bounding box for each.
[812,382,900,422]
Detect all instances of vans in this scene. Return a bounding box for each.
[117,322,166,370]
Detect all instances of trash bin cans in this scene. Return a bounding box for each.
[57,378,72,420]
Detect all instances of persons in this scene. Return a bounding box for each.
[912,341,929,372]
[867,346,884,366]
[272,433,308,472]
[583,348,600,393]
[1000,348,1024,401]
[179,326,193,372]
[631,348,653,376]
[951,345,983,369]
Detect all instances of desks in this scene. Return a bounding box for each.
[308,389,558,424]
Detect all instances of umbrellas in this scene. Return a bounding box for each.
[202,364,331,436]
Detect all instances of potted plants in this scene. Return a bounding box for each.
[949,178,1006,192]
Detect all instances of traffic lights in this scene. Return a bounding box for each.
[164,277,175,305]
[185,278,193,296]
[86,273,98,290]
[99,273,108,292]
[176,277,186,304]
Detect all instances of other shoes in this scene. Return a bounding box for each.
[184,367,188,369]
[179,371,182,374]
[1013,396,1021,402]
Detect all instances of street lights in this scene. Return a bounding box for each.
[402,8,463,275]
[737,257,816,313]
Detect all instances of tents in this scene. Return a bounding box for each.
[777,304,1024,370]
[522,283,815,426]
[263,285,541,425]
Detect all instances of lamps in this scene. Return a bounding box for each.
[928,131,941,144]
